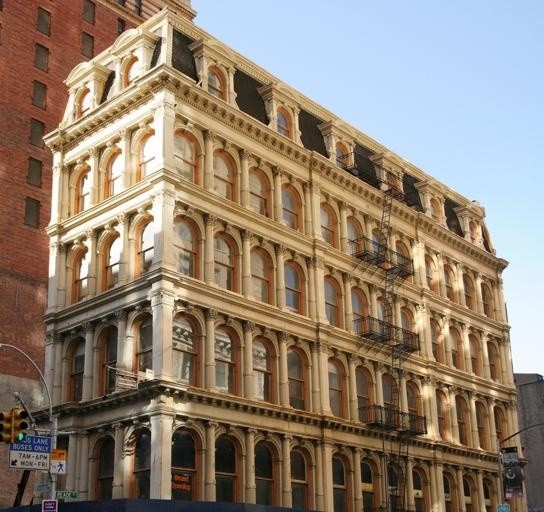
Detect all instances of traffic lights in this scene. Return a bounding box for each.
[0,410,11,442]
[12,409,28,442]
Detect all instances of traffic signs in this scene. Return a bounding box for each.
[50,449,66,475]
[9,434,51,471]
[36,484,50,492]
[54,490,79,499]
[497,503,510,512]
